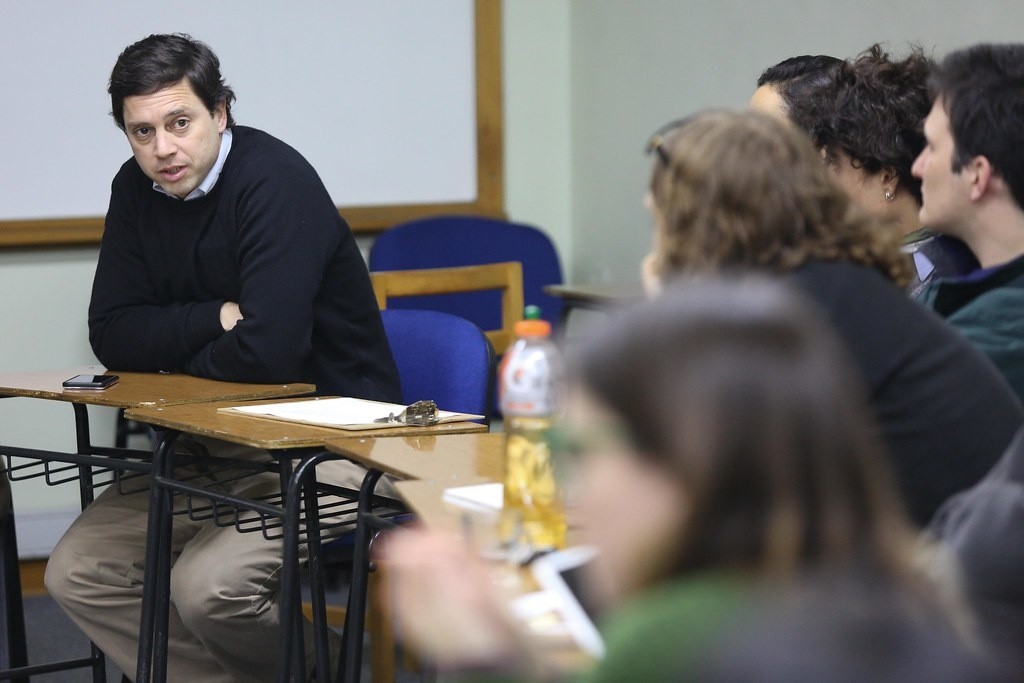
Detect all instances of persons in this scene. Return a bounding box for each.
[372,42,1024,682]
[44,33,410,683]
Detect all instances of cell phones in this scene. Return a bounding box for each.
[63,374,119,387]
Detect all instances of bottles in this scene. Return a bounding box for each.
[498,318,568,551]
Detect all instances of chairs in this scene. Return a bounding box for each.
[300,214,564,683]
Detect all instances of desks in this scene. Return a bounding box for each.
[0,365,317,683]
[324,431,513,683]
[118,395,490,683]
